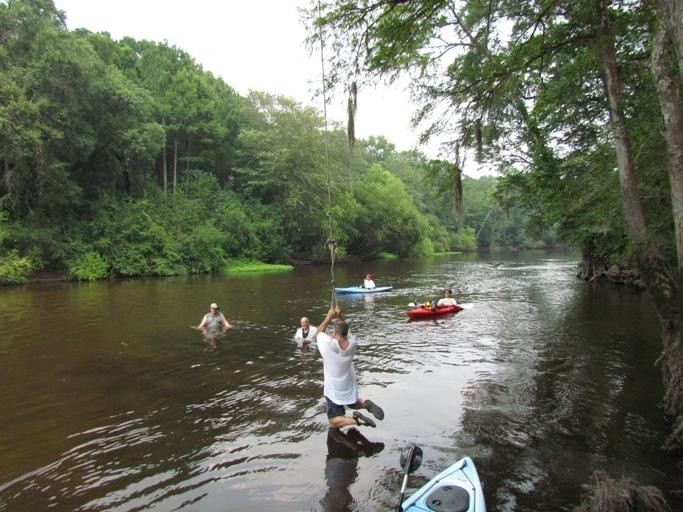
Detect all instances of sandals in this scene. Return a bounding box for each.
[364,400,384,420]
[353,411,376,428]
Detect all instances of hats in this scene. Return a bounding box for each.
[211,303,221,308]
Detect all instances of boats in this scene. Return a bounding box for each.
[333,285,393,295]
[406,304,464,320]
[397,455,487,512]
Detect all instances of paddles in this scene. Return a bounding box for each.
[390,443,423,512]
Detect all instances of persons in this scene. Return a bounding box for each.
[437,288,457,308]
[363,273,376,288]
[294,316,317,341]
[319,426,386,512]
[198,326,225,352]
[197,301,229,329]
[363,294,376,314]
[297,342,317,353]
[317,306,385,429]
